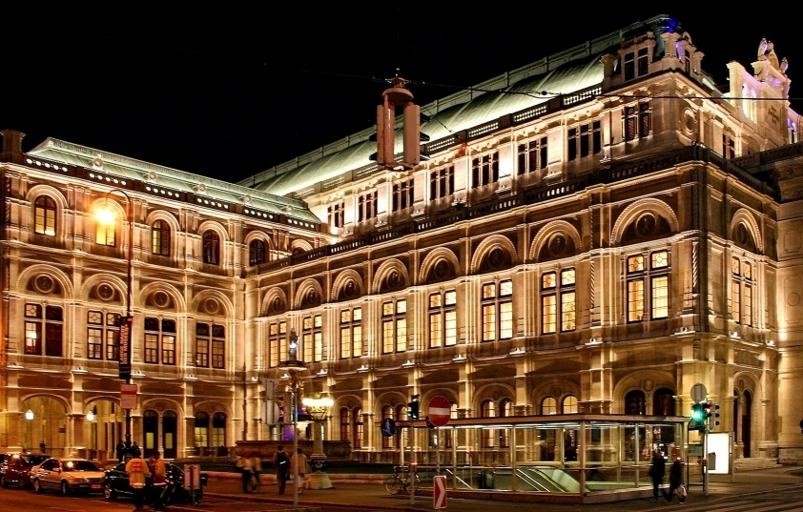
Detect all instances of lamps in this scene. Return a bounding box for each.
[26,399,34,420]
[86,409,94,422]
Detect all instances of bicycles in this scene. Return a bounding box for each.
[383,465,420,495]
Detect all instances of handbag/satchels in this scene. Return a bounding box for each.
[675,486,688,500]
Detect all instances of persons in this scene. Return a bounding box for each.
[116,438,124,462]
[251,450,262,487]
[271,443,290,496]
[124,446,152,510]
[236,454,256,494]
[664,448,688,506]
[645,448,669,500]
[131,440,141,459]
[290,446,310,494]
[147,448,169,512]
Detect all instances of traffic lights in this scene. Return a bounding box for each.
[368,102,429,172]
[689,400,720,430]
[380,418,395,437]
[405,394,419,420]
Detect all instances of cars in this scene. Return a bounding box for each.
[0,450,202,508]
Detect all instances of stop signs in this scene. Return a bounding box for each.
[427,396,450,426]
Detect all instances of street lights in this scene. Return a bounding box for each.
[277,327,307,506]
[89,189,134,457]
[300,398,334,454]
[24,408,34,449]
[86,410,94,449]
